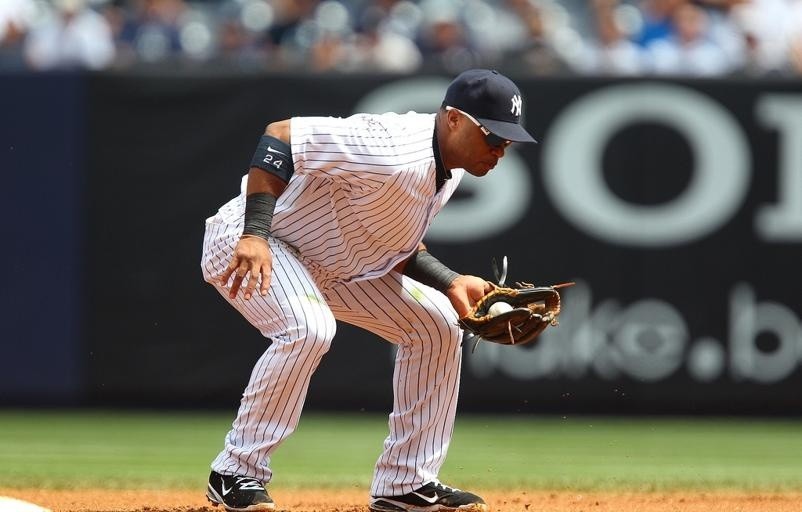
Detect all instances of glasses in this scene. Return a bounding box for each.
[445,105,508,148]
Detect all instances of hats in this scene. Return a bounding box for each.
[446,70,538,144]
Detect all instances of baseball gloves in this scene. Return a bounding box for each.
[458,282,561,344]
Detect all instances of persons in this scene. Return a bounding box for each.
[1,1,800,84]
[196,67,539,511]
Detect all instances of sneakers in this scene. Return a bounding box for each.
[206,471,276,511]
[369,479,487,512]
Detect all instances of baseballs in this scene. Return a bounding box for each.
[488,300,512,315]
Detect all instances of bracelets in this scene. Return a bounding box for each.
[241,191,278,240]
[401,248,463,295]
